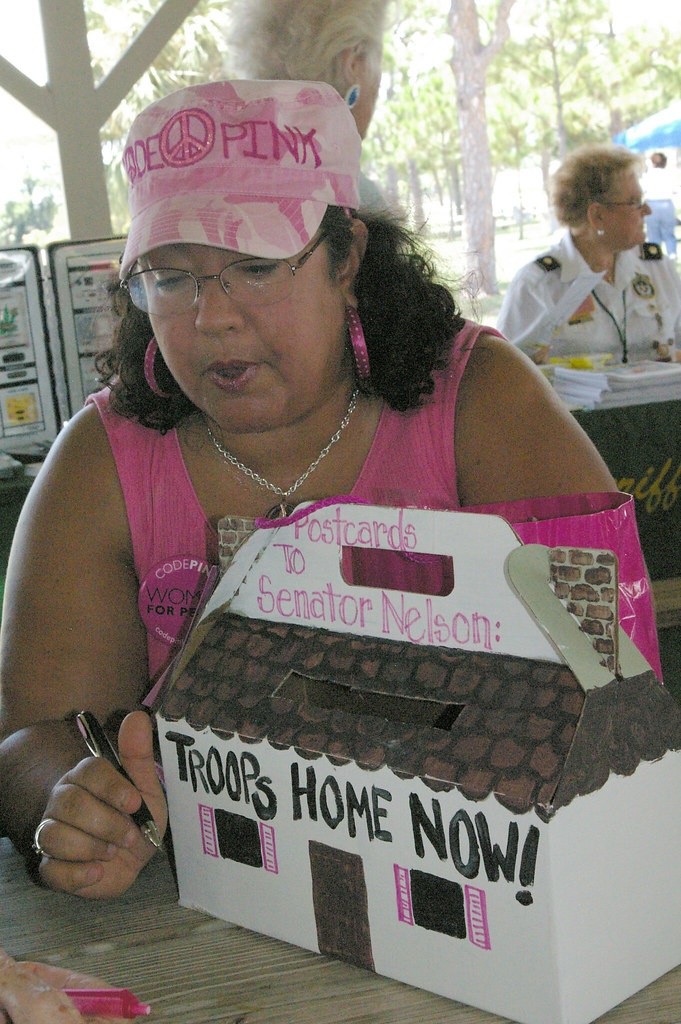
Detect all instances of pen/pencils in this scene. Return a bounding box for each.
[75,709,165,852]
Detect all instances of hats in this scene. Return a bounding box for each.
[119,79,361,280]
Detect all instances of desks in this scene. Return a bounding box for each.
[550,398,680,630]
[1,833,679,1024]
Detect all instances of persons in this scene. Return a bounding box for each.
[498,146,681,364]
[1,79,624,898]
[638,151,680,261]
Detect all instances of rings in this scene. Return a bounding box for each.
[30,817,57,860]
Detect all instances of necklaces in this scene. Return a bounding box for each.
[208,383,360,520]
[592,289,627,364]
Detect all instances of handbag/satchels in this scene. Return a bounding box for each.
[256,491,665,688]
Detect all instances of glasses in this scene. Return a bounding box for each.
[120,229,332,316]
[600,193,646,211]
